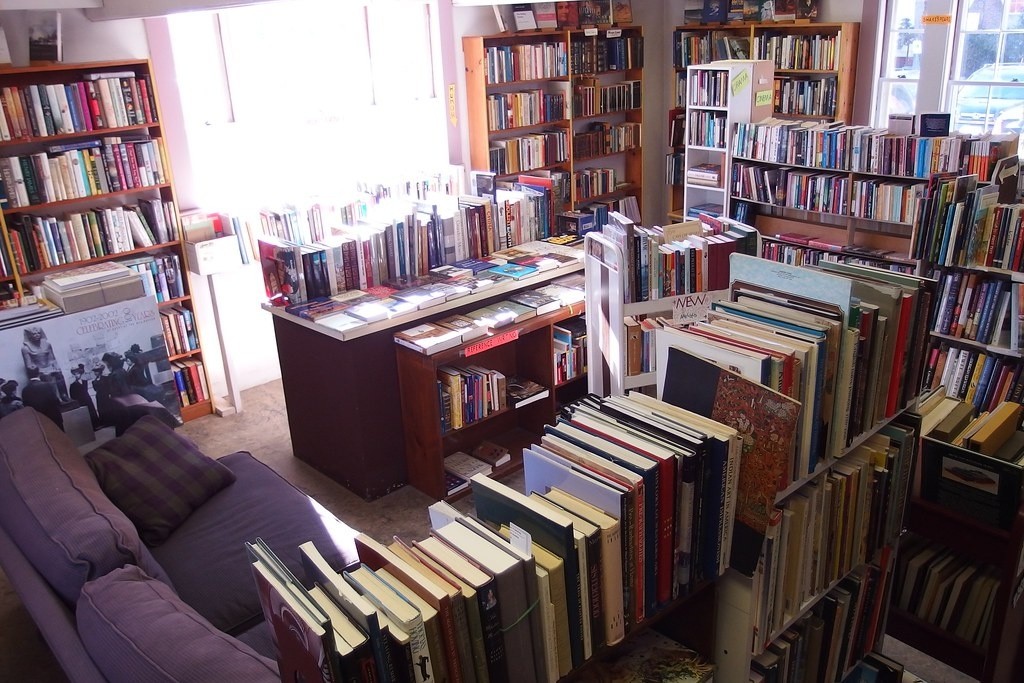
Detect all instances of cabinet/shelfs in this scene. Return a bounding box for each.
[0,51,214,431]
[258,1,1024,683]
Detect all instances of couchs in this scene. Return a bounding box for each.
[0,407,370,683]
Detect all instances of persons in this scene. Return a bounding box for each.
[21,327,70,404]
[0,345,147,429]
[555,3,569,24]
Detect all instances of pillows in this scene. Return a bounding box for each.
[80,413,238,550]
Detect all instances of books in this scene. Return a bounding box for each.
[243,0,1024,683]
[0,69,209,405]
